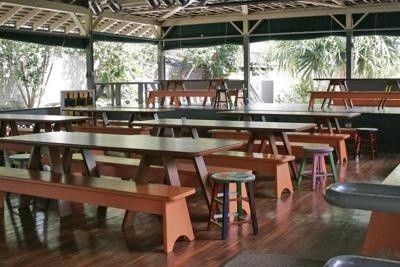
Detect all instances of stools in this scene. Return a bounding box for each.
[214,89,233,110]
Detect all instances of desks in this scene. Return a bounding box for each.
[154,79,228,106]
[313,78,400,110]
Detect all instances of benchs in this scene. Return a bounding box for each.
[0,91,400,254]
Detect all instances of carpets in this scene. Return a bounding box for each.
[219,249,326,267]
